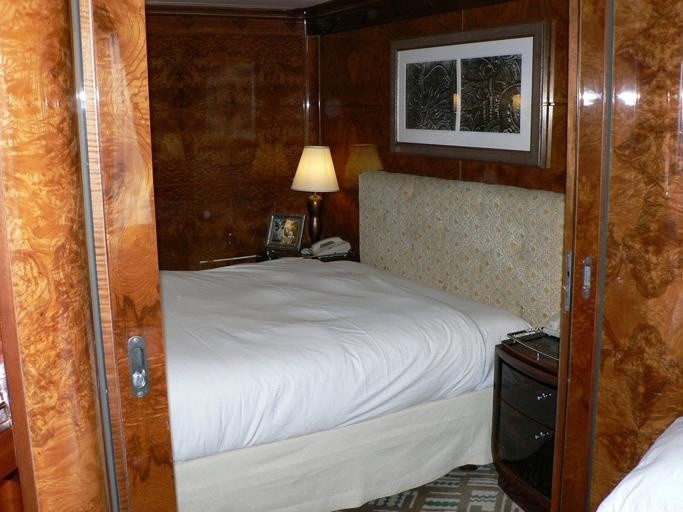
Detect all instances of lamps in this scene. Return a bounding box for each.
[289,145,341,242]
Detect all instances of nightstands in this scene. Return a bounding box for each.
[491,327,563,512]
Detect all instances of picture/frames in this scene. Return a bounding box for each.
[388,19,550,170]
[264,212,306,250]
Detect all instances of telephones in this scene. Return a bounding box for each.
[309,236,351,257]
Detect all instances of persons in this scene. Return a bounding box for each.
[272,221,284,241]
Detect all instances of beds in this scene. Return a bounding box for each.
[161,169,564,510]
